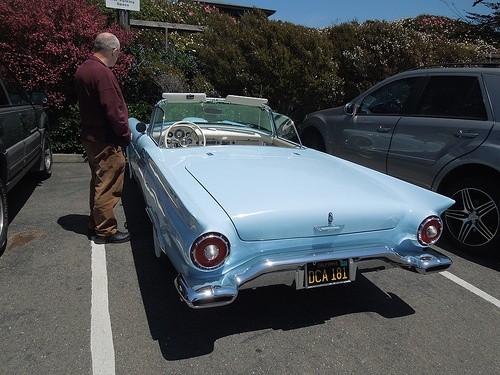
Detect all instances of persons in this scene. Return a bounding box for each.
[74,33,132,244]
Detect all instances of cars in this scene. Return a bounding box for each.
[0,57,53,256]
[127,92,457,310]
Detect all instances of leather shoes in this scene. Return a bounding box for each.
[89,230,132,244]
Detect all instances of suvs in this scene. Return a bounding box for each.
[296,62,500,256]
